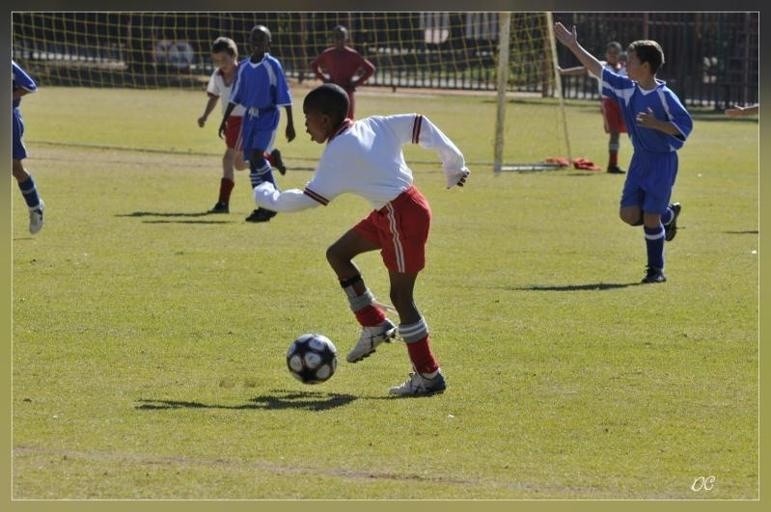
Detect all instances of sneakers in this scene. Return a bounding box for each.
[389,373,446,395]
[606,165,627,175]
[29,199,45,234]
[346,317,398,364]
[206,201,229,214]
[641,272,667,284]
[271,149,286,176]
[663,202,681,241]
[245,207,278,223]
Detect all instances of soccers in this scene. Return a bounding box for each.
[287,335,338,384]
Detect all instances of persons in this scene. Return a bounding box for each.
[306,24,378,126]
[11,60,48,236]
[551,20,696,286]
[196,35,288,216]
[555,39,632,177]
[217,24,299,226]
[249,78,474,402]
